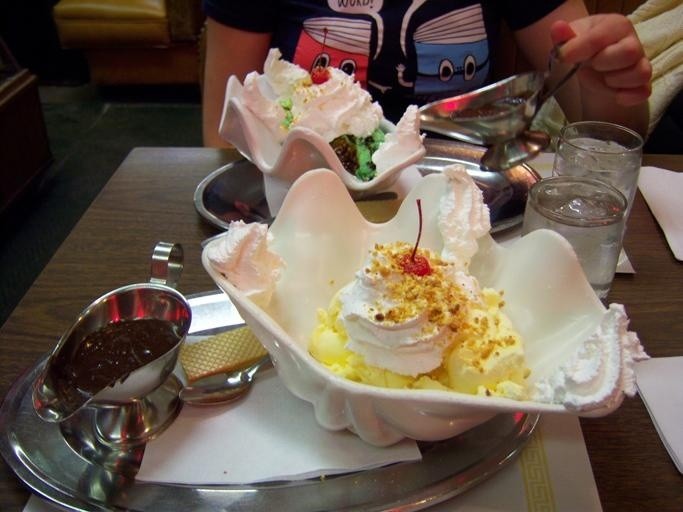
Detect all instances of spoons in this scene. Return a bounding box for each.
[180,356,269,407]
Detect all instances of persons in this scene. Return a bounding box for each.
[197,3,657,156]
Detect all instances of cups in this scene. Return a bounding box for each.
[415,48,580,172]
[513,121,643,299]
[32,242,193,451]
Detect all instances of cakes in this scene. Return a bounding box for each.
[241,46,426,182]
[206,163,650,413]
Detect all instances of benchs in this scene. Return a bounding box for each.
[50,0,203,86]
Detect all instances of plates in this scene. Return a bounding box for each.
[220,67,421,201]
[198,166,622,453]
